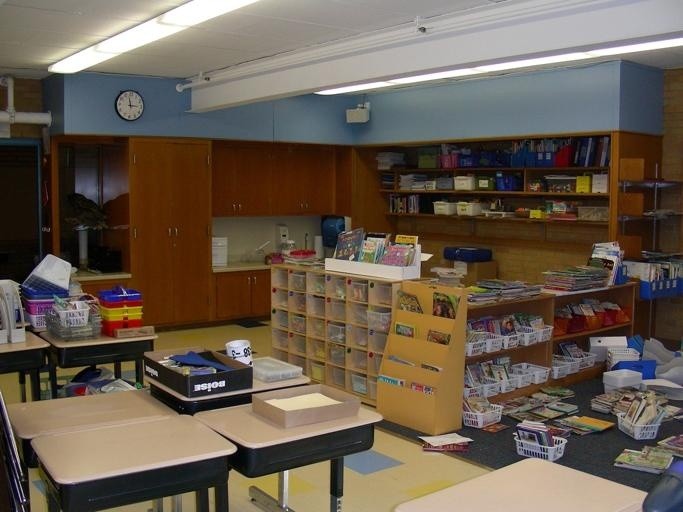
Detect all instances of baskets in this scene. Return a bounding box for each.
[21,284,71,300]
[22,254,71,295]
[464,304,660,462]
[69,294,97,315]
[22,295,73,315]
[96,285,144,337]
[24,301,90,329]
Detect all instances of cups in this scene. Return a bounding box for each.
[226,340,253,367]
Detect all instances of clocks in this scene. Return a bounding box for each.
[116,91,144,120]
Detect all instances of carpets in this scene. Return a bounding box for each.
[375,381,683,493]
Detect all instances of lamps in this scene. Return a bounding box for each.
[47,0,260,74]
[414,16,428,35]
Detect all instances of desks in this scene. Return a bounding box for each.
[0,328,51,398]
[42,328,157,389]
[392,457,649,512]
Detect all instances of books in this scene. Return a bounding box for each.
[493,383,580,422]
[465,356,513,395]
[441,143,458,167]
[376,151,393,169]
[388,194,420,213]
[558,341,589,368]
[411,383,433,395]
[613,449,673,475]
[548,415,616,438]
[400,173,427,191]
[590,392,682,439]
[463,396,495,427]
[432,293,460,318]
[623,249,683,291]
[560,298,631,331]
[422,441,469,452]
[396,291,422,313]
[422,362,443,372]
[333,229,419,268]
[395,324,415,337]
[378,172,395,190]
[388,356,415,367]
[379,375,405,386]
[590,241,625,285]
[468,311,551,351]
[467,278,539,303]
[516,419,554,460]
[482,423,510,432]
[540,265,608,292]
[431,266,464,290]
[427,329,451,344]
[504,139,610,168]
[657,434,683,458]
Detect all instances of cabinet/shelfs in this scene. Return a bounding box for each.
[211,269,270,322]
[555,282,637,387]
[211,139,272,218]
[100,136,210,324]
[377,280,468,436]
[463,293,555,404]
[335,144,392,234]
[270,142,338,217]
[378,130,663,224]
[39,137,130,299]
[618,180,682,264]
[270,264,392,406]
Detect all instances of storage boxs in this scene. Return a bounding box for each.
[458,202,486,216]
[453,176,474,189]
[437,177,454,190]
[433,200,456,216]
[143,347,252,416]
[440,260,497,286]
